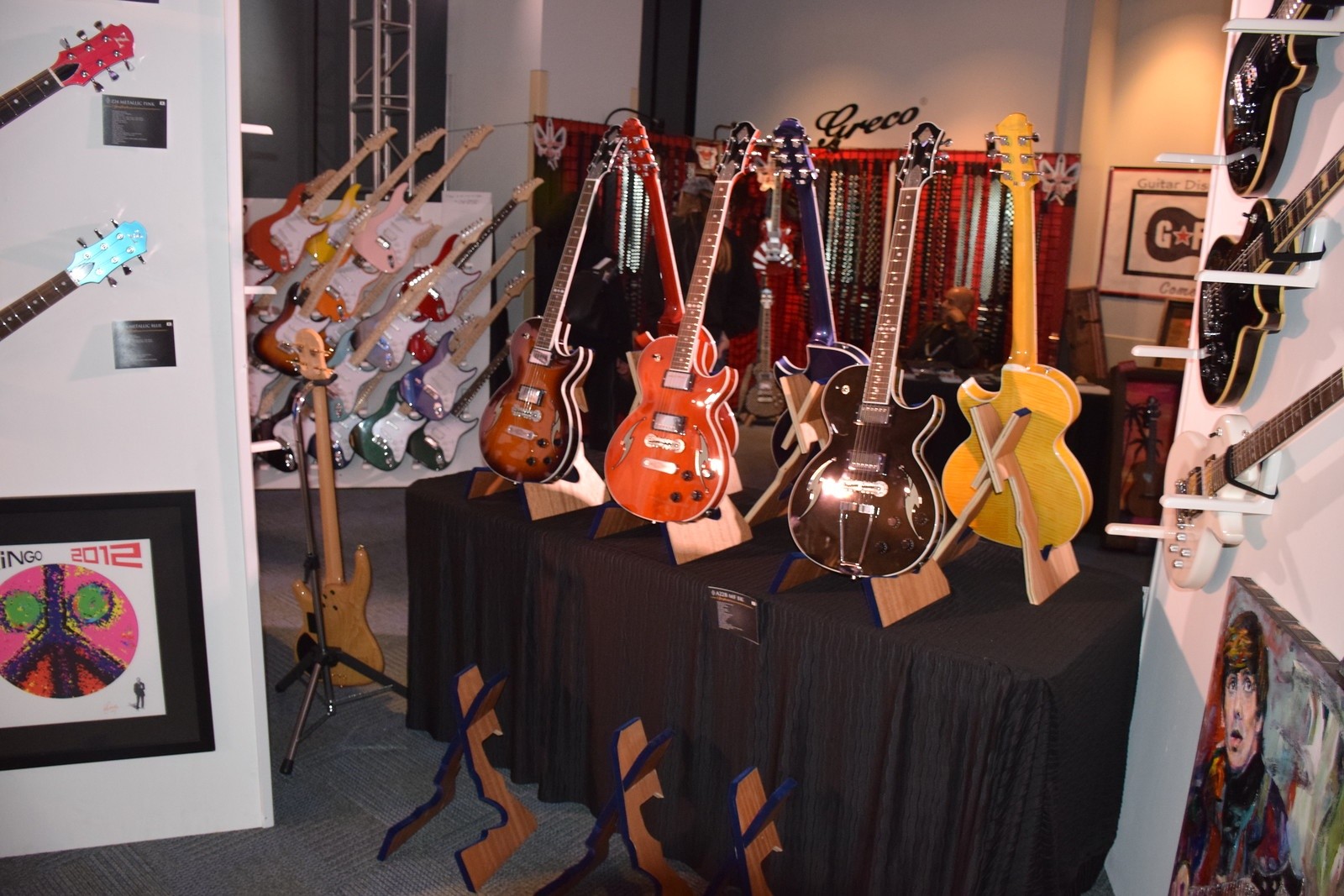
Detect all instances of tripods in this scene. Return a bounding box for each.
[272,375,407,774]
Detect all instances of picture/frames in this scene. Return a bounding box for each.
[1094,167,1214,301]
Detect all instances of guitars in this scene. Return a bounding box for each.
[1222,0,1344,200]
[479,124,627,485]
[285,328,386,687]
[622,117,738,457]
[767,116,871,473]
[940,112,1096,549]
[246,123,545,476]
[1161,369,1344,594]
[1197,146,1344,408]
[603,118,769,524]
[786,120,953,577]
[0,19,136,131]
[0,218,149,343]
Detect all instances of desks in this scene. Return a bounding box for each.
[380,476,1151,894]
